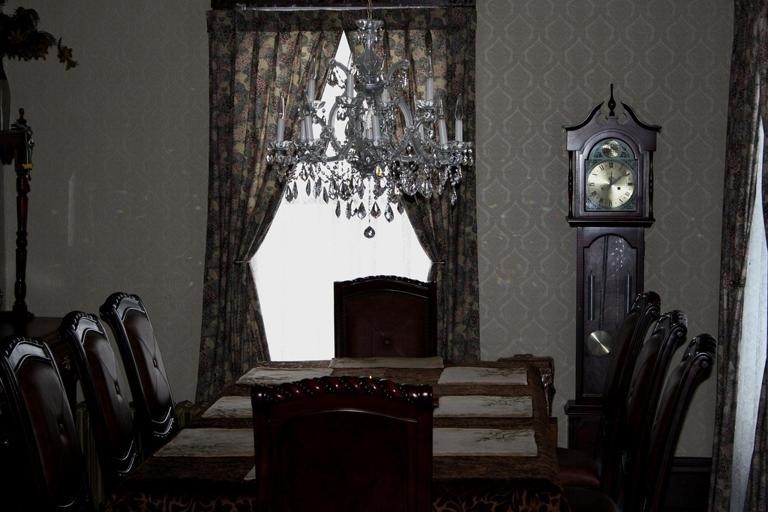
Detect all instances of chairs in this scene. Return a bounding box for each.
[59,308,142,512]
[332,274,440,359]
[612,289,660,401]
[0,336,91,511]
[100,292,177,455]
[556,333,719,511]
[625,308,688,433]
[246,378,441,511]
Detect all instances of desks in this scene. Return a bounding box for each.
[128,357,555,509]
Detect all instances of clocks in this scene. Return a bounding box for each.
[562,80,664,457]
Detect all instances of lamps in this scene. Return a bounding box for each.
[265,17,477,239]
